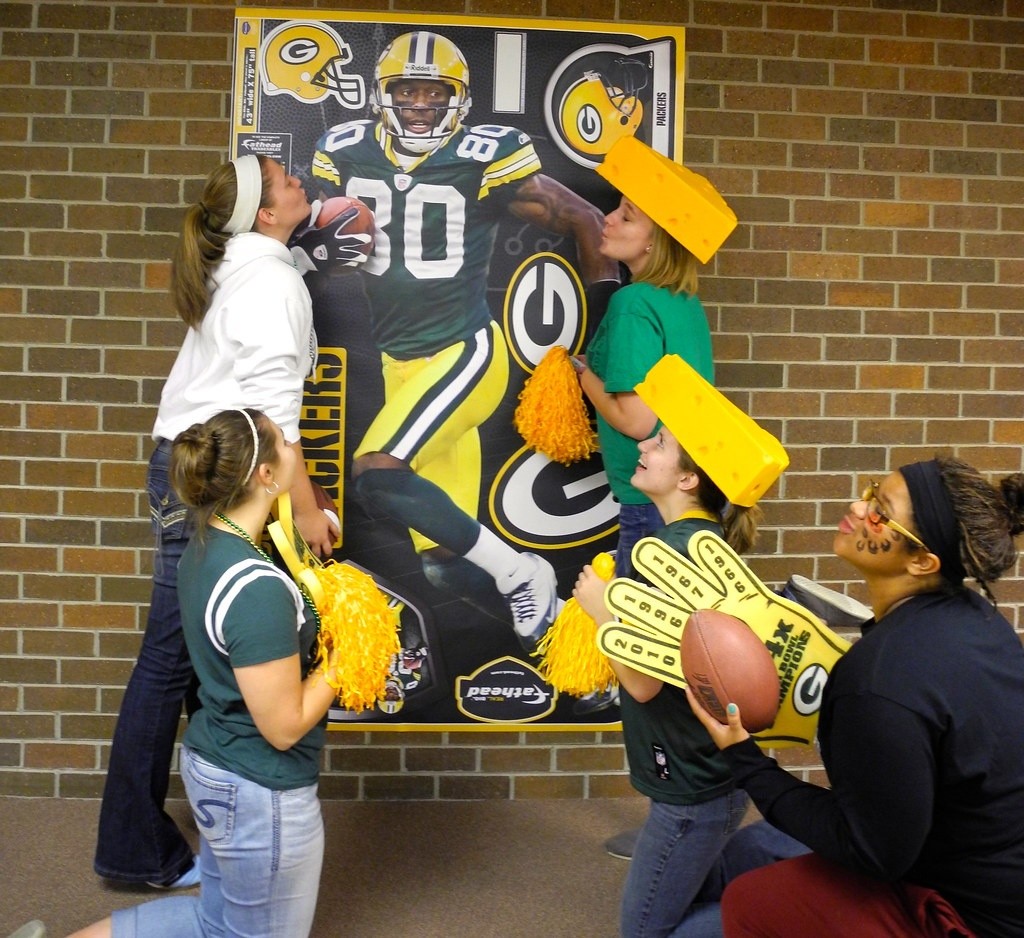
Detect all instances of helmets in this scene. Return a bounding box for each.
[369,31,474,153]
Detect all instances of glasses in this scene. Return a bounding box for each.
[862,478,931,553]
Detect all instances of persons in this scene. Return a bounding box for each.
[93,155,340,891]
[569,136,876,859]
[287,32,621,722]
[686,456,1024,938]
[573,355,817,938]
[6,410,344,938]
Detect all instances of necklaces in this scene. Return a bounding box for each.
[213,511,321,638]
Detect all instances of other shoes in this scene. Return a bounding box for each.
[145,852,203,889]
[604,823,642,862]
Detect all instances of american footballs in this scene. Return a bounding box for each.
[681,610,780,733]
[319,196,375,273]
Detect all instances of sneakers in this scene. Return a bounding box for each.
[500,551,558,651]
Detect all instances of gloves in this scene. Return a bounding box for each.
[596,527,852,746]
[583,280,621,352]
[286,199,373,278]
